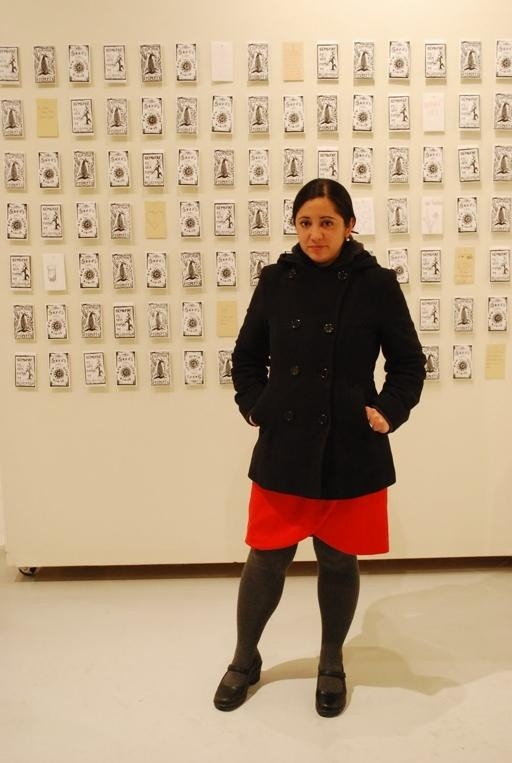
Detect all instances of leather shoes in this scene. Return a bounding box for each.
[315,664,346,716]
[215,651,262,710]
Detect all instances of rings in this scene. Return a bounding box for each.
[367,422,375,429]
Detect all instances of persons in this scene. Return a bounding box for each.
[210,179,427,718]
